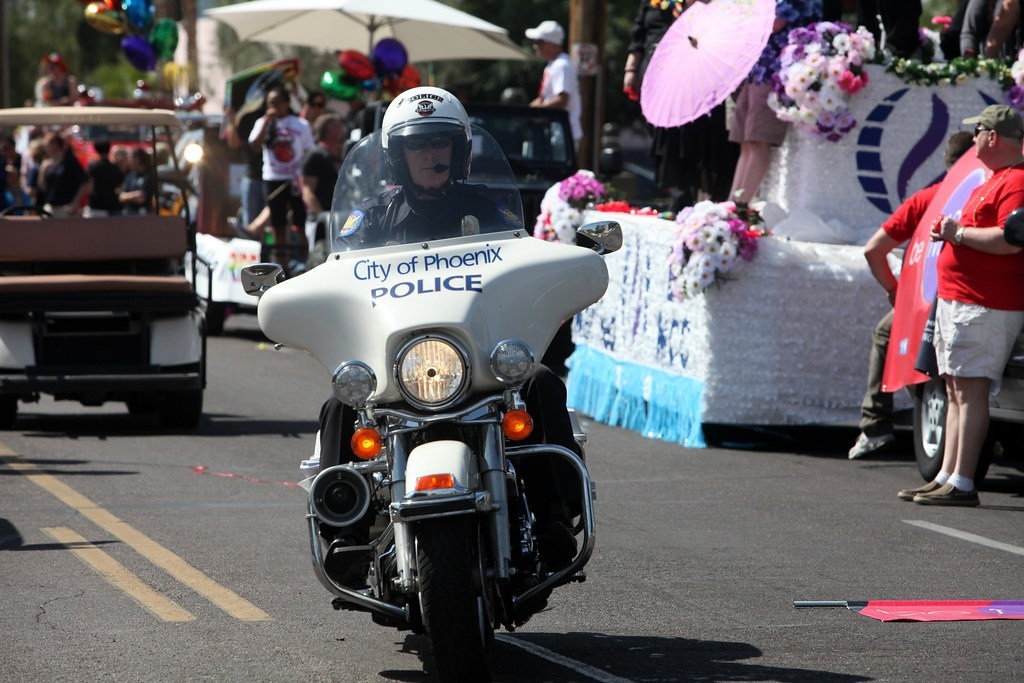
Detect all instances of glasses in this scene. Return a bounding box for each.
[975,126,991,137]
[401,135,450,150]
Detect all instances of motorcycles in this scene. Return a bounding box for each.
[231,114,624,667]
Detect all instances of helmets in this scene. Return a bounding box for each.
[382,86,472,186]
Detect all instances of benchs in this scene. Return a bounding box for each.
[0,214,191,293]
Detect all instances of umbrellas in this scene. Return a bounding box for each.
[639,0,777,130]
[204,0,532,62]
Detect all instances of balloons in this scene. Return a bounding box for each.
[319,38,420,98]
[84,0,178,90]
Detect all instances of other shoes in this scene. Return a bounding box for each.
[848,431,895,459]
[897,479,980,506]
[962,104,1024,139]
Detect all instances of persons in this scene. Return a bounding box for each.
[621,0,1024,225]
[898,104,1024,506]
[0,54,388,275]
[288,86,590,592]
[848,131,973,460]
[525,20,584,201]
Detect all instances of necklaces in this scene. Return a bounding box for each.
[960,160,1021,228]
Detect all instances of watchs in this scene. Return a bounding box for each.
[955,227,965,246]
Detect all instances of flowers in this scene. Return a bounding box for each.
[534,169,603,243]
[673,194,768,300]
[760,15,1024,141]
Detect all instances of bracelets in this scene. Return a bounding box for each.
[624,68,635,72]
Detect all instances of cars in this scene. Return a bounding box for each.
[446,103,582,245]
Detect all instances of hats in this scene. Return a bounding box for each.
[525,20,564,46]
